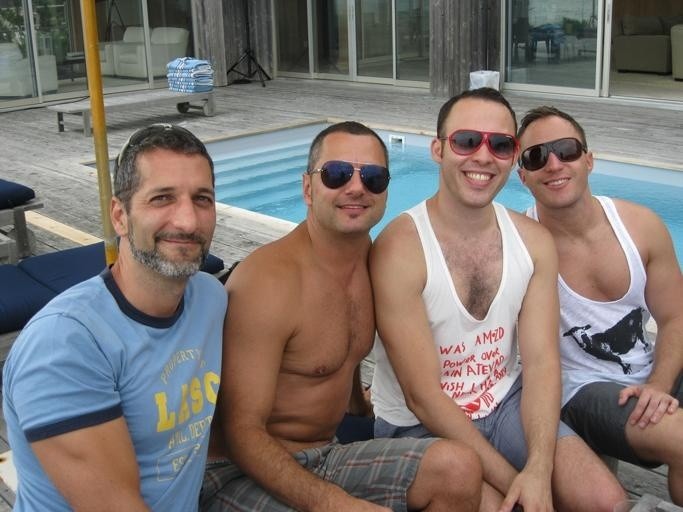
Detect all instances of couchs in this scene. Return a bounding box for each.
[611,14,683,81]
[113,27,190,78]
[98,26,151,75]
[0,43,58,96]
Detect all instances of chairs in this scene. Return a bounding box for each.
[1,236,226,339]
[1,179,43,258]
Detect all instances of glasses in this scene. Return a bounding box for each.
[309,160,392,194]
[520,137,588,171]
[118,123,194,166]
[440,128,520,161]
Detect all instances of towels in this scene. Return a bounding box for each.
[166,57,214,93]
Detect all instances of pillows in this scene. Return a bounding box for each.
[623,14,663,35]
[660,16,683,34]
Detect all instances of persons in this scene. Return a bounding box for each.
[367,87,632,512]
[515,106,683,507]
[1,123,229,512]
[200,122,482,512]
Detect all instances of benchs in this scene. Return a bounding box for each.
[46,86,216,137]
